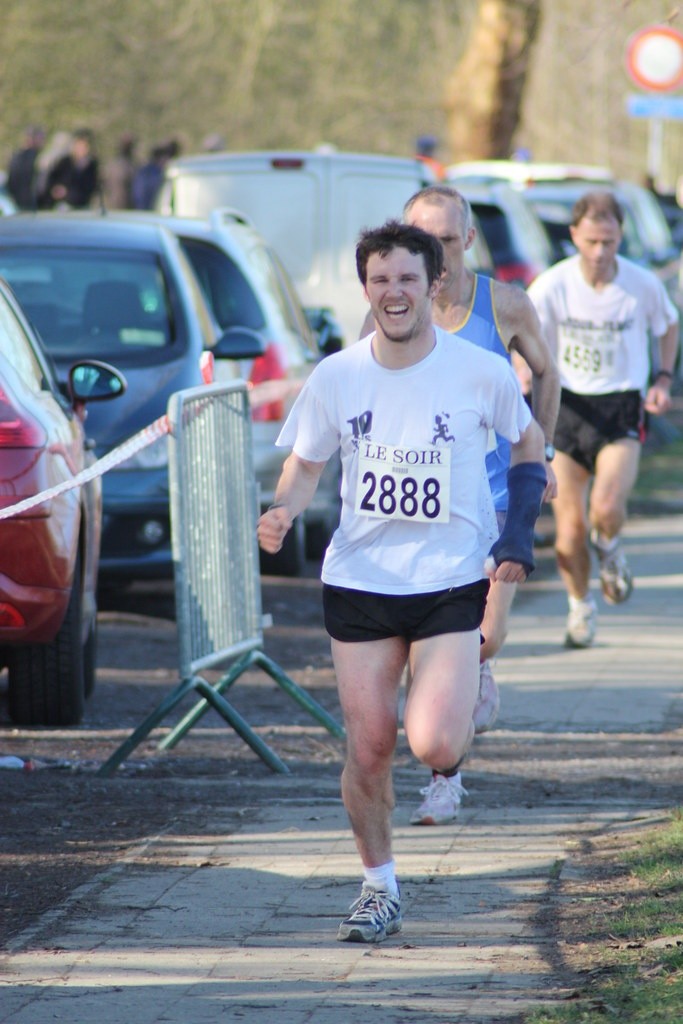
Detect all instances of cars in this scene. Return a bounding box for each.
[102,206,347,578]
[1,273,128,728]
[419,156,683,350]
[2,208,277,598]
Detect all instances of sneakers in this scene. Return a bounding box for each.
[472,659,500,733]
[409,770,469,825]
[589,527,632,604]
[567,596,596,647]
[337,875,402,942]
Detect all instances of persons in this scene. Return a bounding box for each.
[256,220,548,943]
[508,192,681,648]
[361,185,562,825]
[7,127,100,211]
[108,133,179,210]
[414,133,446,179]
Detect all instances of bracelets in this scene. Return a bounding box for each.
[658,369,672,379]
[268,504,282,510]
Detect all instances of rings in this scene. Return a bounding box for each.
[484,562,497,570]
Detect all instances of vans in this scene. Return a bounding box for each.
[152,152,492,433]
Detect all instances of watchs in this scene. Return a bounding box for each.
[545,443,554,461]
